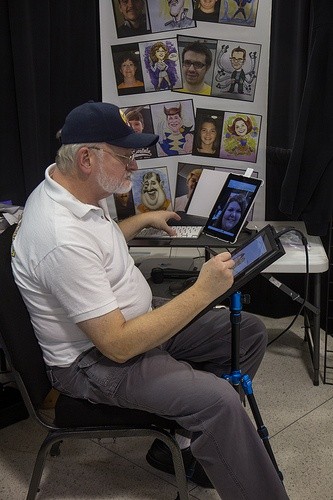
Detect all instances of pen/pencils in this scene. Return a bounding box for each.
[202,247,219,257]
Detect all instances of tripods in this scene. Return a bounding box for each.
[176,291,283,500]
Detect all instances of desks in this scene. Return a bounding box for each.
[129,221,330,386]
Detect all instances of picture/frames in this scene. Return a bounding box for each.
[201,172,264,243]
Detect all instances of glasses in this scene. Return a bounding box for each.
[88,146,135,162]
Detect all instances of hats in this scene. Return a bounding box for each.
[60,100,160,149]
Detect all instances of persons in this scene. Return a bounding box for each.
[126,110,158,161]
[193,117,221,156]
[117,53,144,88]
[171,42,213,95]
[193,0,220,23]
[210,194,247,235]
[173,168,203,212]
[10,100,292,500]
[117,0,152,37]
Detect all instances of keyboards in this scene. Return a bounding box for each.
[133,226,205,239]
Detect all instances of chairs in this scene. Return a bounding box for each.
[1,266,188,499]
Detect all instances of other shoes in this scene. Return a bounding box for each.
[145,438,215,490]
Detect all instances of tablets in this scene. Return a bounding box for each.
[230,228,278,279]
[201,173,264,244]
